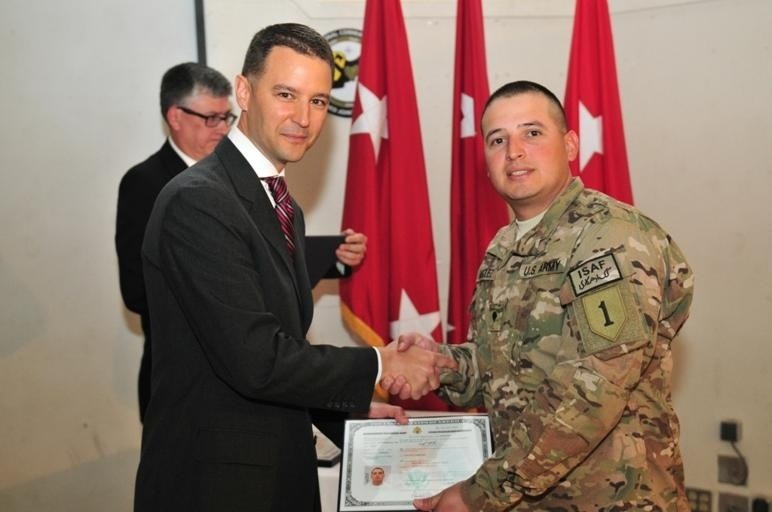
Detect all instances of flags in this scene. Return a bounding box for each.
[562,0,632,206]
[339,1,448,413]
[448,1,512,413]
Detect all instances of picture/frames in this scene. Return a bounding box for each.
[336,413,496,511]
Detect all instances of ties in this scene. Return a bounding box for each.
[263,176,295,271]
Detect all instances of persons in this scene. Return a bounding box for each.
[379,80,693,512]
[367,468,386,486]
[115,62,368,426]
[132,23,460,512]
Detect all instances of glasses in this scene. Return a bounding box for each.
[178,105,236,127]
